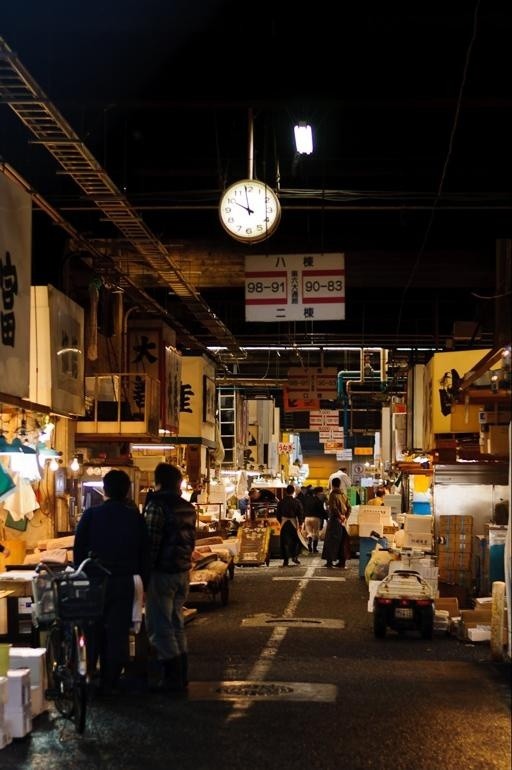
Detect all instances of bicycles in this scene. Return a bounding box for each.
[31,551,112,744]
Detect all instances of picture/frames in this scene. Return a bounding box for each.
[161,344,182,434]
[203,374,217,423]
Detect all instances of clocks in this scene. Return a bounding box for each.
[219,179,281,244]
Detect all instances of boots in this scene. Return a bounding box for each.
[334,556,346,567]
[283,556,289,565]
[325,559,333,567]
[149,652,189,691]
[307,537,318,553]
[292,546,300,563]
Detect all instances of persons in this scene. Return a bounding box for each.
[135,461,197,695]
[243,466,353,569]
[70,468,144,686]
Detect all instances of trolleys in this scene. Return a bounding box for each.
[233,502,275,566]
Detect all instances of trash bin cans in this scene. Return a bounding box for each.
[412,502,431,515]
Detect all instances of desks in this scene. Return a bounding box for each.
[6,563,76,648]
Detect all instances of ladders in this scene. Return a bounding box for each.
[218,386,236,471]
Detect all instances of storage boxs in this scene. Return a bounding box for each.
[0,643,48,749]
[450,404,484,433]
[478,426,510,462]
[347,505,512,644]
[196,540,241,555]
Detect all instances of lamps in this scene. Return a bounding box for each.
[0,409,81,471]
